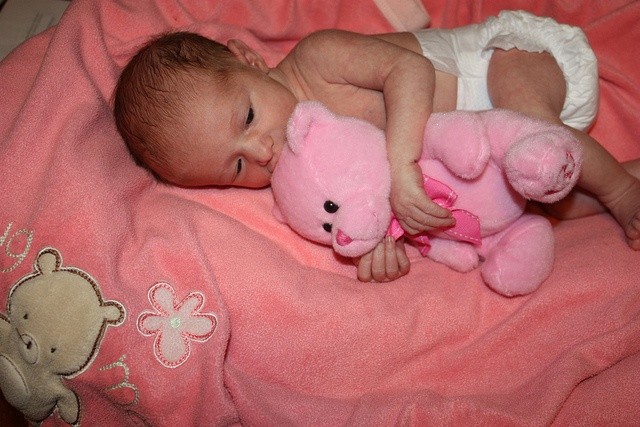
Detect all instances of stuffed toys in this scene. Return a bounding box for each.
[271,101,581,299]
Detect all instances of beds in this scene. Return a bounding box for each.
[2,3,639,424]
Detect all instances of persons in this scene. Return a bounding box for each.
[114,30,636,283]
